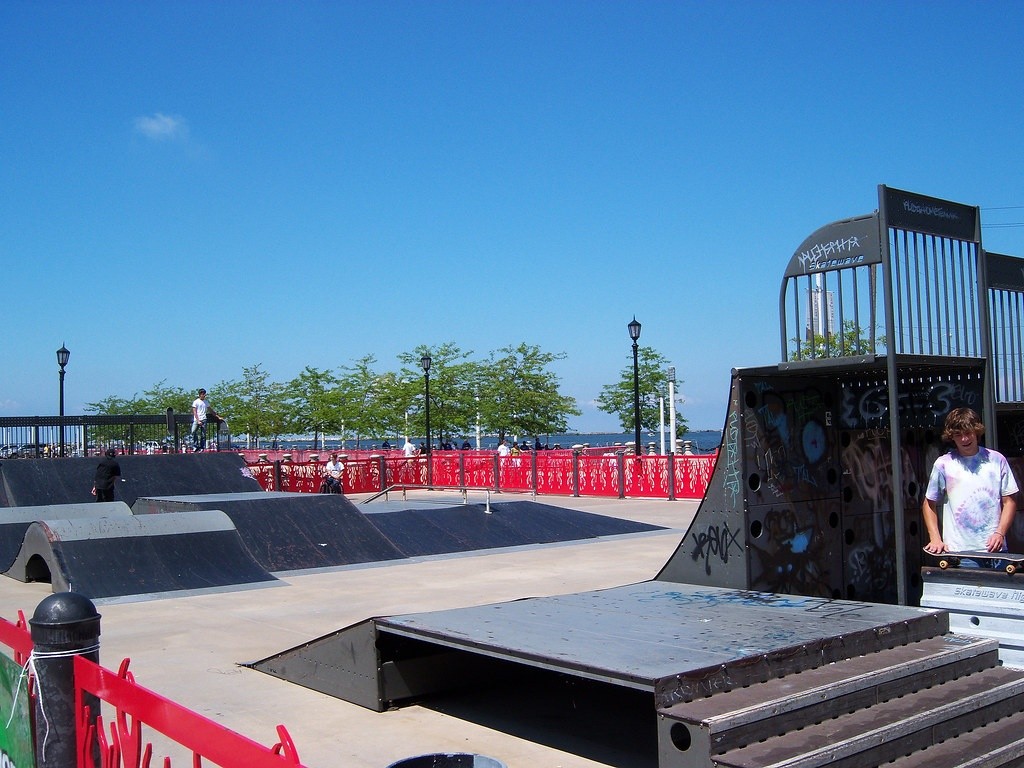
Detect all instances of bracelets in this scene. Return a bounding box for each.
[994,531,1004,539]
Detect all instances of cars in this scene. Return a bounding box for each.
[0,433,217,459]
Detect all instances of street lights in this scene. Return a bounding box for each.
[627,314,642,455]
[57,342,72,459]
[419,351,435,492]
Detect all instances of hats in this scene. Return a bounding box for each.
[106,449,114,459]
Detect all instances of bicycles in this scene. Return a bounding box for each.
[318,472,343,495]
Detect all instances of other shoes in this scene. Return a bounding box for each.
[198,448,204,454]
[191,447,198,453]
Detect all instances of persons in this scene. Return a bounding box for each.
[146,443,154,454]
[324,452,345,494]
[162,442,167,454]
[497,440,510,471]
[438,439,457,451]
[510,442,523,483]
[91,449,122,502]
[520,441,529,450]
[44,443,56,458]
[417,442,427,467]
[535,438,542,450]
[922,408,1019,568]
[182,443,186,453]
[169,443,174,453]
[461,439,471,450]
[401,438,416,471]
[542,445,548,450]
[191,388,223,453]
[382,438,391,455]
